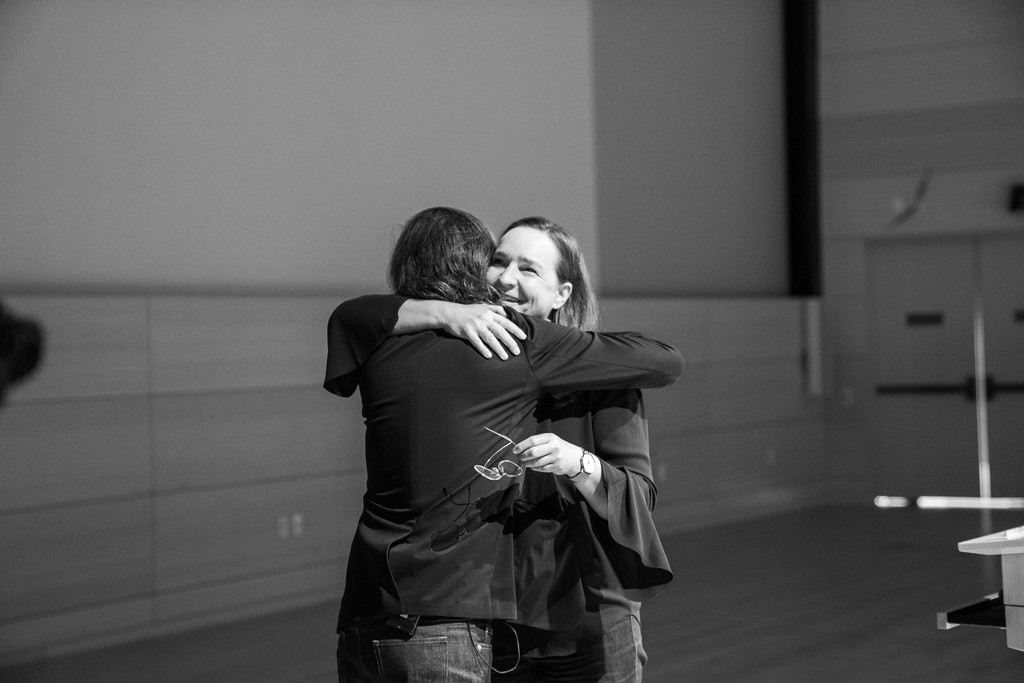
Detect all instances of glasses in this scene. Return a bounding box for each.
[471,423,528,482]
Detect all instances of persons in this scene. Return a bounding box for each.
[322,214,675,683]
[333,206,685,682]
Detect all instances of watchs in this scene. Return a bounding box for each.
[568,446,595,483]
